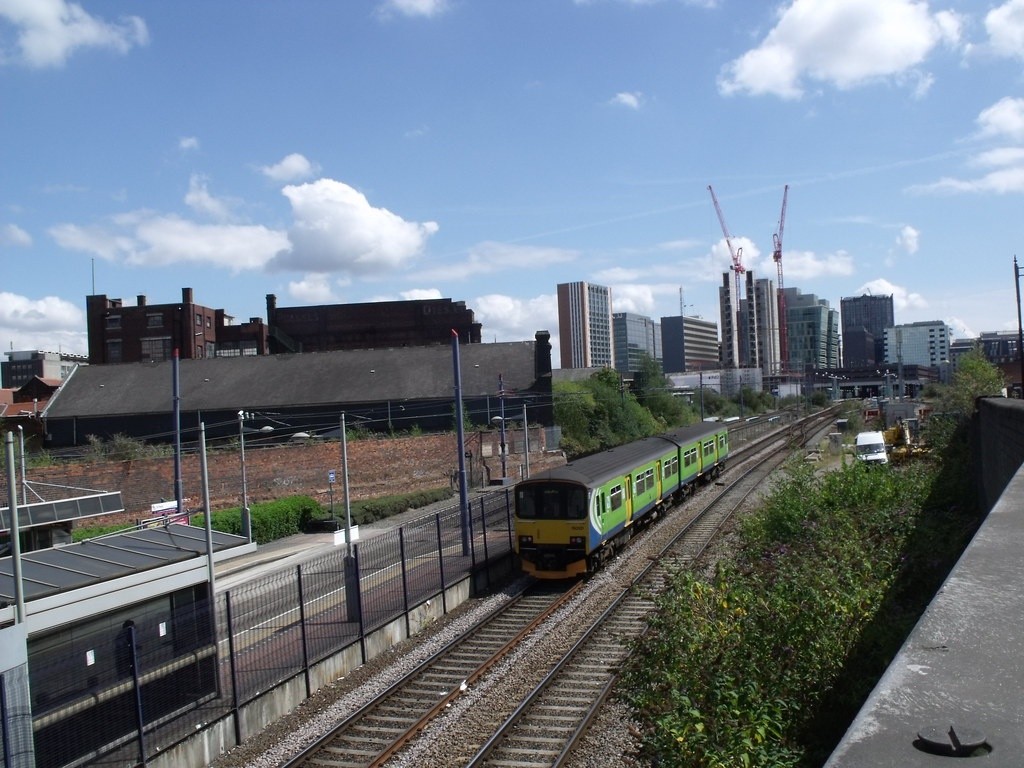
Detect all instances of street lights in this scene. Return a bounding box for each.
[237,411,274,544]
[291,411,360,623]
[491,404,530,478]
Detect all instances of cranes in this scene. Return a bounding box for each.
[706,186,757,365]
[772,184,796,372]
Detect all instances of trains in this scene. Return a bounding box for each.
[513,422,730,580]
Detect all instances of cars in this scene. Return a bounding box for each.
[854,431,888,466]
